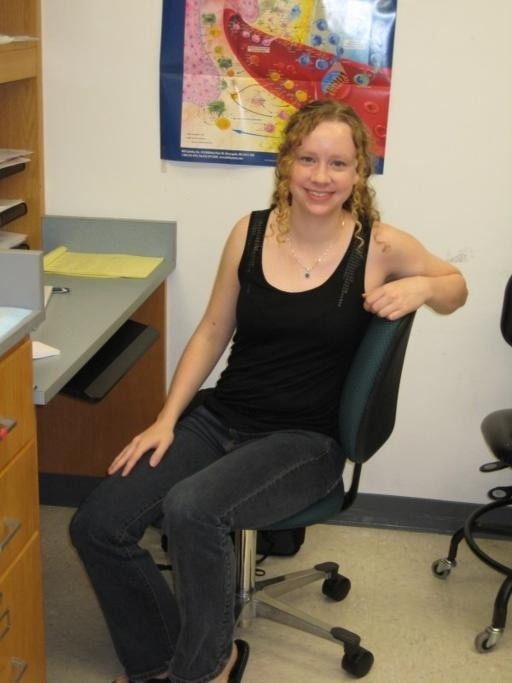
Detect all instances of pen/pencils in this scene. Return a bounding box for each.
[52,288,69,293]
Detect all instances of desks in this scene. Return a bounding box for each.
[1,214,178,682]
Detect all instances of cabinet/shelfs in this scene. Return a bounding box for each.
[0,0,43,250]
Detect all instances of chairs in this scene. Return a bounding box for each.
[162,294,418,679]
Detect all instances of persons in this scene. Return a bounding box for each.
[67,96,471,683]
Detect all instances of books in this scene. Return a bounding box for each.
[43,244,165,280]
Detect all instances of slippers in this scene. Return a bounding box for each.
[227,638,250,683]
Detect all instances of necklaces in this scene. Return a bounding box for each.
[284,216,342,280]
